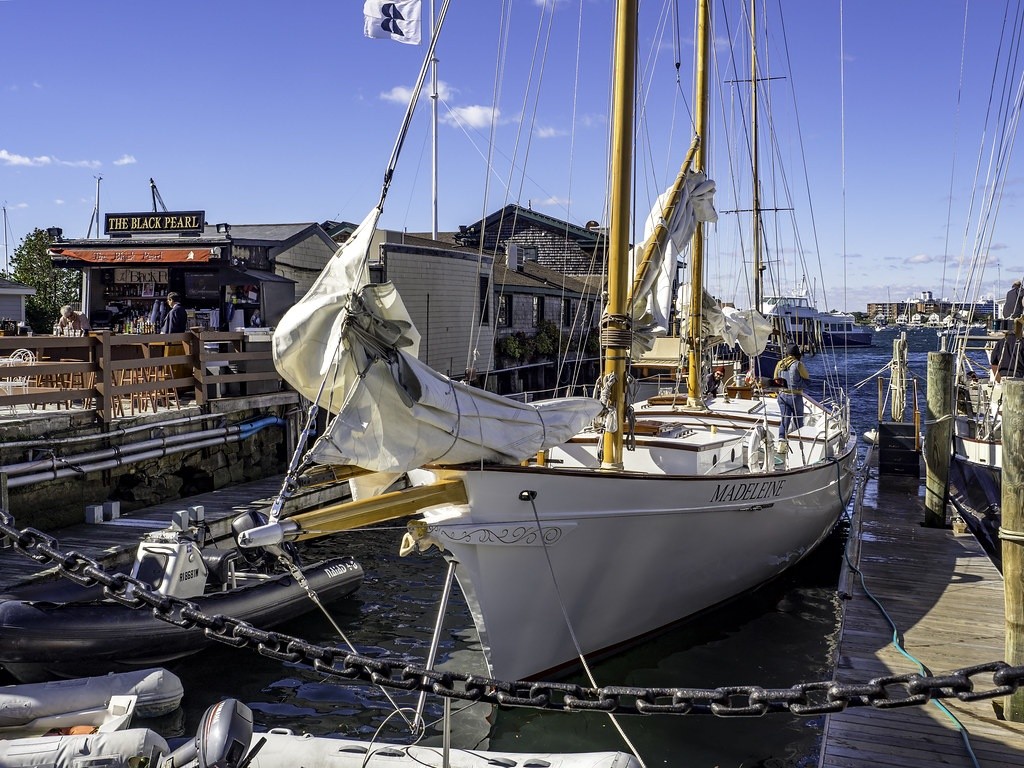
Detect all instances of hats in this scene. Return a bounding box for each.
[786,343,800,356]
[713,366,725,379]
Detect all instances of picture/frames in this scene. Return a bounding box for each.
[142,282,155,297]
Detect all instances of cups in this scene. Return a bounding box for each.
[63,327,81,336]
[27,332,32,337]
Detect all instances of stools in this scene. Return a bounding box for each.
[34,357,180,419]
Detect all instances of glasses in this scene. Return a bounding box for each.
[66,312,73,319]
[715,372,724,377]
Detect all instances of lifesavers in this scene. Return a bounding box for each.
[747,426,775,475]
[971,373,978,382]
[955,389,968,405]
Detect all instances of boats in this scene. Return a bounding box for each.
[752,273,872,349]
[0,510,365,680]
[0,666,184,768]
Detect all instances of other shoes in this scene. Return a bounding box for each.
[777,438,788,454]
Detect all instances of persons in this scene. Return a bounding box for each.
[160,292,187,400]
[773,342,812,454]
[990,331,1024,384]
[703,366,725,396]
[57,305,92,337]
[1002,280,1024,320]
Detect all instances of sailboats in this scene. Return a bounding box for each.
[235,0,857,707]
[948,0,1024,578]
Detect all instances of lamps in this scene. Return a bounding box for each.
[216,223,232,238]
[48,226,64,243]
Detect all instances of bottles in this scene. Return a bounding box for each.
[104,284,140,297]
[53,317,59,336]
[231,295,236,304]
[119,308,160,334]
[17,320,25,335]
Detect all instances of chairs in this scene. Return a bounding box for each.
[0,348,35,419]
